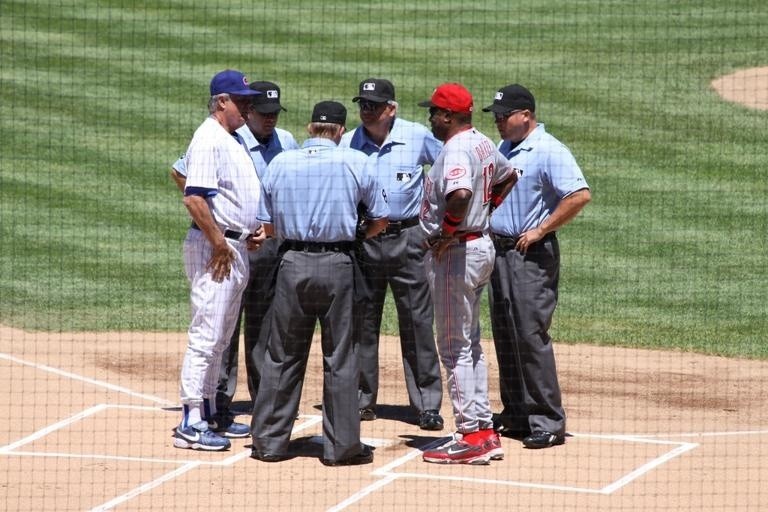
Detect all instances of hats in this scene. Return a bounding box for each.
[418,82,473,114]
[249,81,287,114]
[311,100,347,131]
[352,78,396,106]
[482,83,535,112]
[209,69,262,95]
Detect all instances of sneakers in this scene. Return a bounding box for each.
[173,418,231,452]
[422,429,505,465]
[206,414,249,438]
[414,411,443,430]
[360,406,376,419]
[325,443,373,465]
[493,415,565,448]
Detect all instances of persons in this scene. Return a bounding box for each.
[414,83,520,467]
[481,84,593,451]
[171,68,271,449]
[170,80,300,437]
[251,97,392,468]
[332,76,449,431]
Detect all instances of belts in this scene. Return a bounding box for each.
[423,232,481,249]
[190,217,256,242]
[361,216,421,234]
[282,240,351,254]
